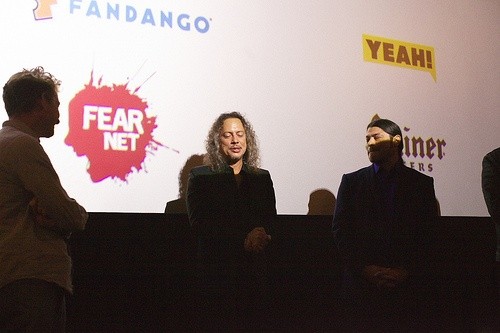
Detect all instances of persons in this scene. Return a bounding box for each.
[481,147,500,228]
[0,66,88,333]
[331,119,441,333]
[185,113,277,333]
